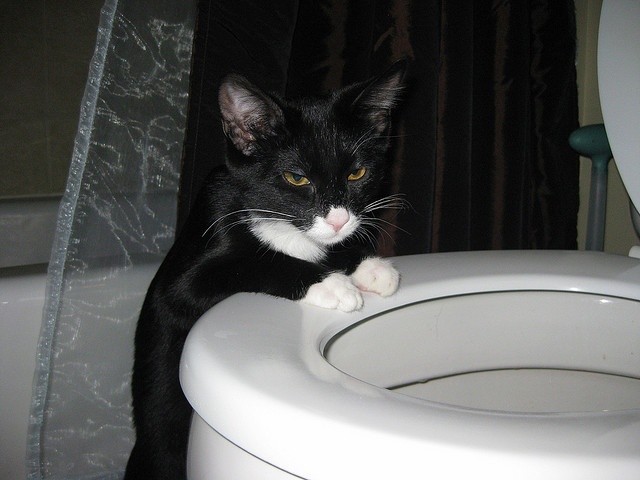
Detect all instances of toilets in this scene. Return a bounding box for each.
[178,0,639,478]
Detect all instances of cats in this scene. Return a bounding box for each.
[121,49,412,480]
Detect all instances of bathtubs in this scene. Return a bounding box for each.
[1,188,193,480]
